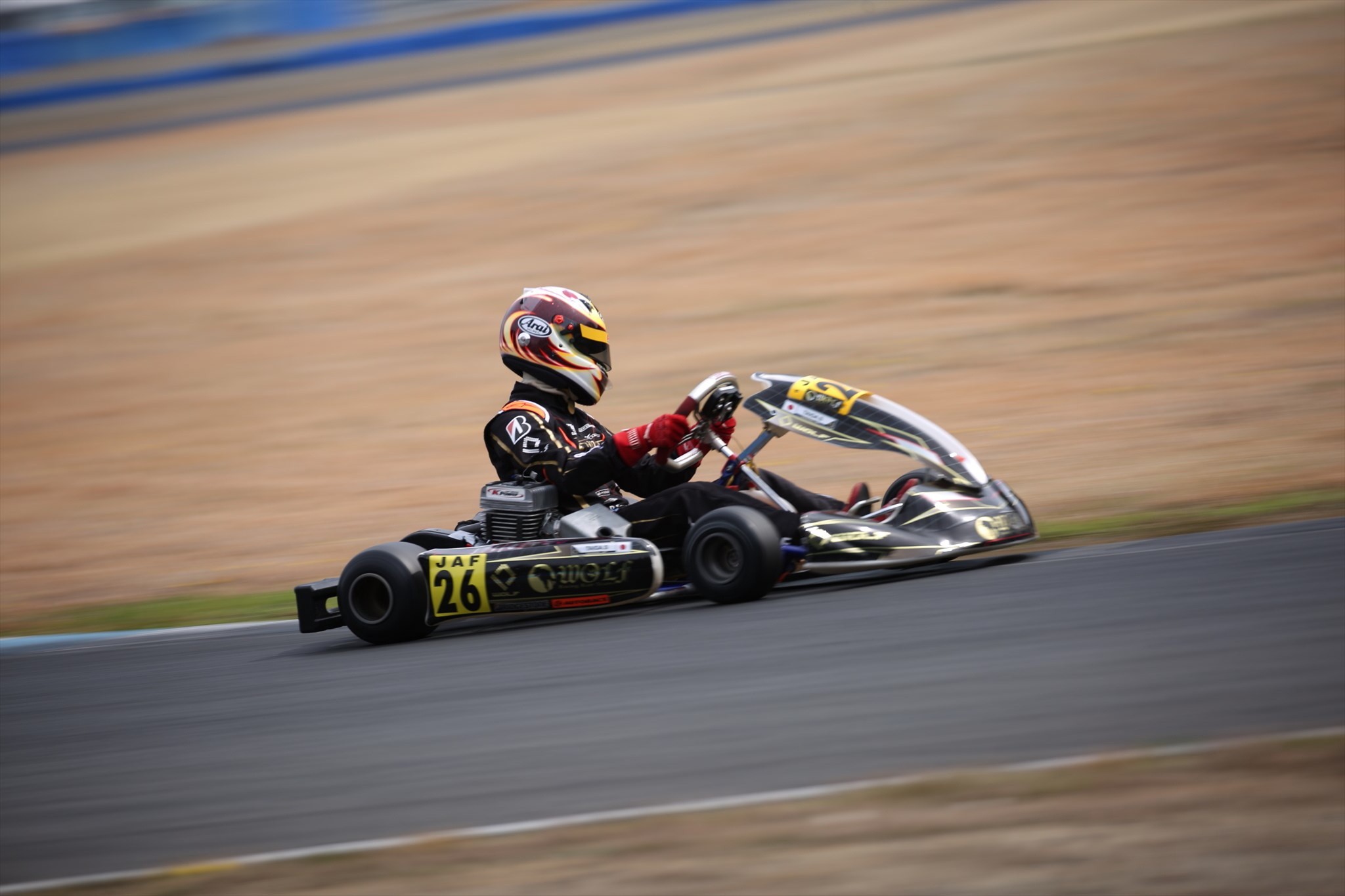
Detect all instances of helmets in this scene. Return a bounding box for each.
[499,286,613,406]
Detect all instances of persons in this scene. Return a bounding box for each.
[483,286,872,545]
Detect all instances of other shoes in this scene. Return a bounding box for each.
[872,478,920,522]
[843,482,871,516]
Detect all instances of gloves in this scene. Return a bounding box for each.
[613,414,691,470]
[676,415,736,467]
